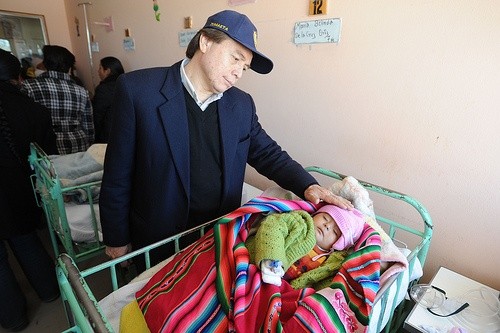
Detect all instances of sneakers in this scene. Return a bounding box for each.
[37,285,60,302]
[11,311,29,331]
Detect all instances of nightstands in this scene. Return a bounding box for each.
[403,265,500,333]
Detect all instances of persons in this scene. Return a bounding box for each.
[246,205,365,290]
[99,10,353,282]
[0,45,124,333]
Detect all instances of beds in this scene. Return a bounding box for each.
[30,140,433,333]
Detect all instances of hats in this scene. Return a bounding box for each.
[43,45,75,67]
[311,204,364,262]
[203,10,273,74]
